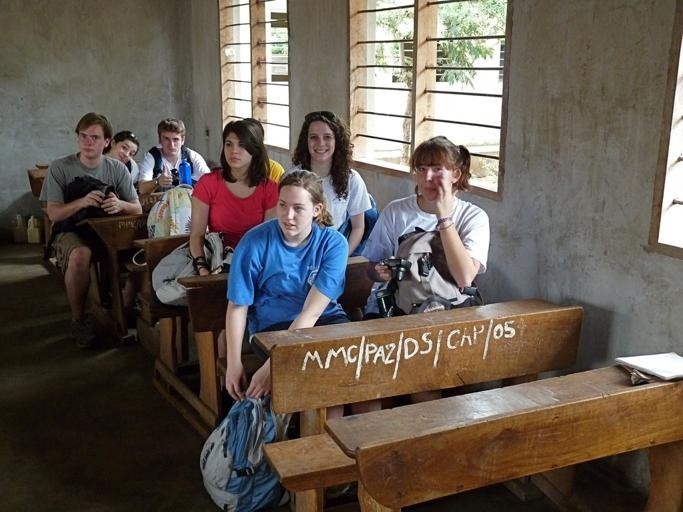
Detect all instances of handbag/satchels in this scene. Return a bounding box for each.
[43,175,119,261]
[375,227,483,319]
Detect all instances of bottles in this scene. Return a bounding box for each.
[177,156,192,186]
[169,169,179,187]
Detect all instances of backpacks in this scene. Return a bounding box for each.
[152,231,235,308]
[146,184,193,239]
[199,389,293,510]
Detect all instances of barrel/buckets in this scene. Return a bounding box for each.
[28,215,43,244]
[11,213,28,243]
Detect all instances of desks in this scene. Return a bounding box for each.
[27,163,683,512]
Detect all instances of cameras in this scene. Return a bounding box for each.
[381,257,413,280]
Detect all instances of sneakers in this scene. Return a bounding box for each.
[70,317,95,349]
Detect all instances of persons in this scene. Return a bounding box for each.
[38,113,143,349]
[244,118,284,184]
[278,111,371,321]
[138,118,211,193]
[361,136,490,403]
[223,169,380,499]
[103,130,139,185]
[190,121,279,412]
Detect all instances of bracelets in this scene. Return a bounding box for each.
[436,217,453,226]
[155,178,159,186]
[438,223,454,230]
[192,256,208,272]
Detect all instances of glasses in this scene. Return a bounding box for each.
[305,110,333,119]
